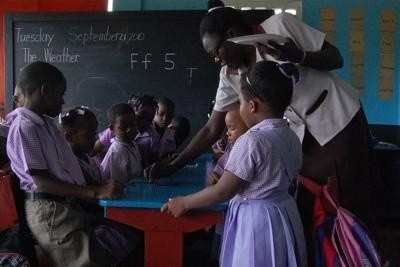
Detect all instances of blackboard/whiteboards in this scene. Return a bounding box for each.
[4,9,275,165]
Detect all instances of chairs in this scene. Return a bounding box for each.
[0,173,53,267]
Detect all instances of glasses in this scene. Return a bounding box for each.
[209,41,224,58]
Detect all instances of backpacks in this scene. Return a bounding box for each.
[321,184,386,267]
[306,186,342,267]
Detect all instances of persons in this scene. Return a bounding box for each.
[143,0,385,251]
[0,58,191,266]
[207,104,249,184]
[161,177,162,179]
[160,60,307,266]
[161,162,162,164]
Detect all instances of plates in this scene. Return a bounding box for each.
[226,32,290,48]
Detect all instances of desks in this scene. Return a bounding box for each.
[372,140,400,191]
[100,153,228,267]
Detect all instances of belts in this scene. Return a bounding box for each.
[22,192,64,200]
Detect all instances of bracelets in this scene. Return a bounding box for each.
[295,50,306,64]
